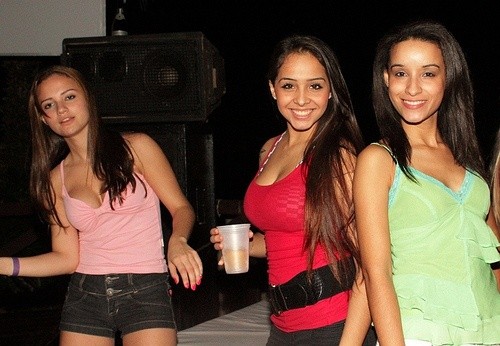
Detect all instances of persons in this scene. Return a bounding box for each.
[351,23,499,346]
[210,38,377,346]
[0,65,202,346]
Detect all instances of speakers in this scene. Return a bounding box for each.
[62,32,227,124]
[113,124,216,250]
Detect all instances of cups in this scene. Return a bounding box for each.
[216,224,251,274]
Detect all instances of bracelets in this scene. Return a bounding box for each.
[9,257,20,278]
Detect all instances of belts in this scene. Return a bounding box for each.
[266,256,356,317]
